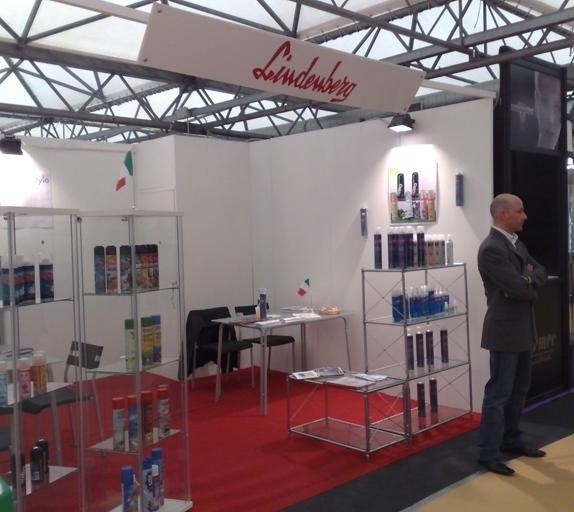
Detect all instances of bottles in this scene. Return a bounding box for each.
[9,437,49,494]
[120,448,165,512]
[94,242,159,295]
[359,203,367,237]
[455,169,464,208]
[0,249,55,306]
[110,384,169,453]
[371,171,453,431]
[257,287,269,321]
[124,314,164,370]
[0,345,48,405]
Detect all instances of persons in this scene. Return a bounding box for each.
[534,72,562,149]
[477,193,549,475]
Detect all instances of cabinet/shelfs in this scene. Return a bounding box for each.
[0,205,84,512]
[359,261,472,436]
[72,212,194,512]
[288,371,407,459]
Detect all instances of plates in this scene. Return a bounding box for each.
[320,310,342,315]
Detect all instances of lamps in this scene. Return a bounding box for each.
[387,115,415,132]
[1,134,24,154]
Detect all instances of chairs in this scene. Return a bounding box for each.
[236,306,295,384]
[179,306,255,389]
[23,342,104,444]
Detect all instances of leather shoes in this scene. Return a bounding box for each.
[478,460,515,476]
[512,446,545,458]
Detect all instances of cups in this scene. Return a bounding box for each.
[254,307,260,320]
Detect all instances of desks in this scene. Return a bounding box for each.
[209,309,353,415]
[8,382,74,465]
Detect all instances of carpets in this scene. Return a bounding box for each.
[0,371,482,511]
